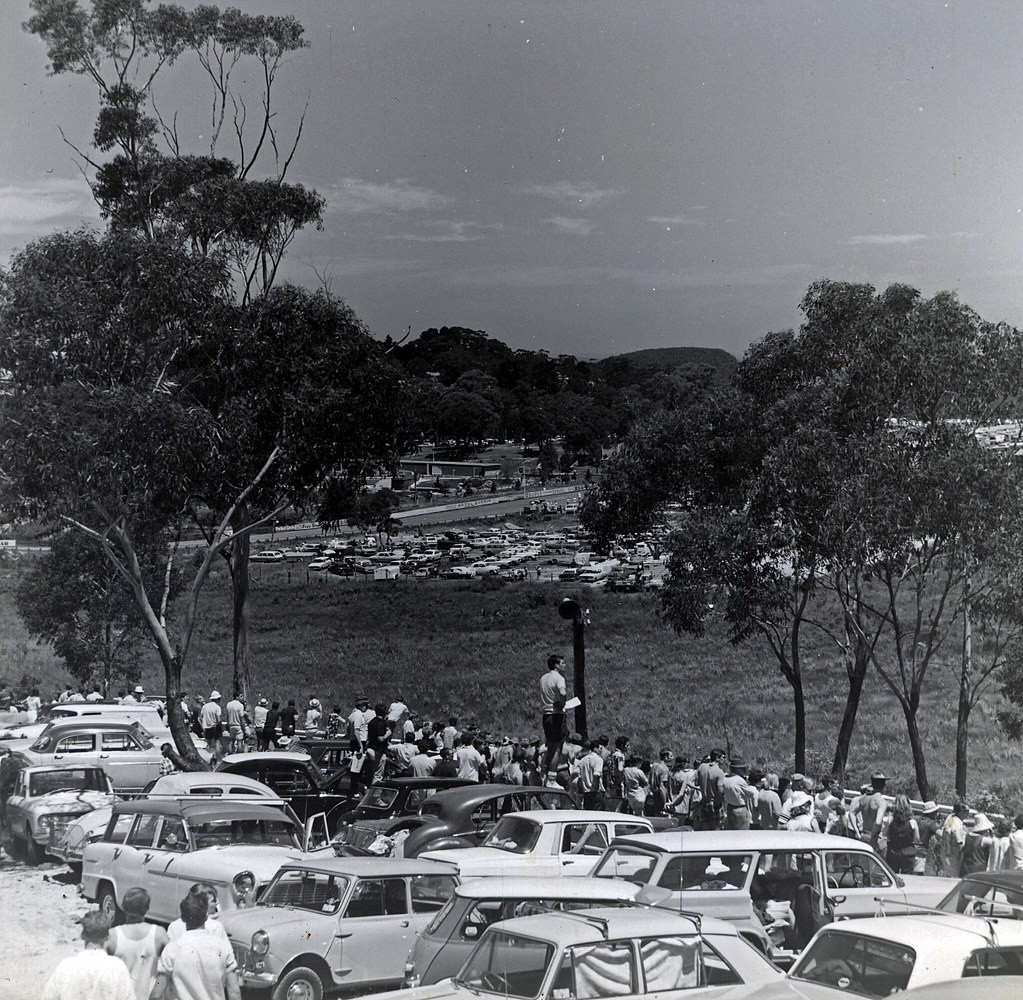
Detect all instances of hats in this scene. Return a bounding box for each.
[259,699,268,705]
[789,791,811,812]
[529,735,539,743]
[290,735,300,741]
[508,737,519,746]
[871,772,891,780]
[502,736,511,744]
[354,695,371,705]
[310,699,319,706]
[972,813,994,832]
[730,756,746,768]
[277,736,291,744]
[135,686,144,692]
[209,690,221,699]
[922,801,939,813]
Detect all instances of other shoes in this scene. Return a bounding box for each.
[374,798,389,806]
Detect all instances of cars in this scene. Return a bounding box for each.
[249,502,692,593]
[0,706,1023,1000]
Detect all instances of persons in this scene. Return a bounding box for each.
[536,565,542,581]
[254,698,299,751]
[237,822,261,843]
[180,690,253,760]
[572,496,576,504]
[345,695,1023,875]
[562,823,596,854]
[324,709,348,742]
[509,567,527,581]
[148,883,241,1000]
[304,698,322,740]
[682,856,795,930]
[554,500,558,510]
[105,888,170,1000]
[0,682,164,723]
[539,655,568,789]
[567,497,570,504]
[37,911,136,1000]
[159,743,180,776]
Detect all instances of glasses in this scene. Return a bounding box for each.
[967,810,969,812]
[137,693,143,694]
[806,801,811,805]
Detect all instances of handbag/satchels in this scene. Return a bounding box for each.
[348,751,366,773]
